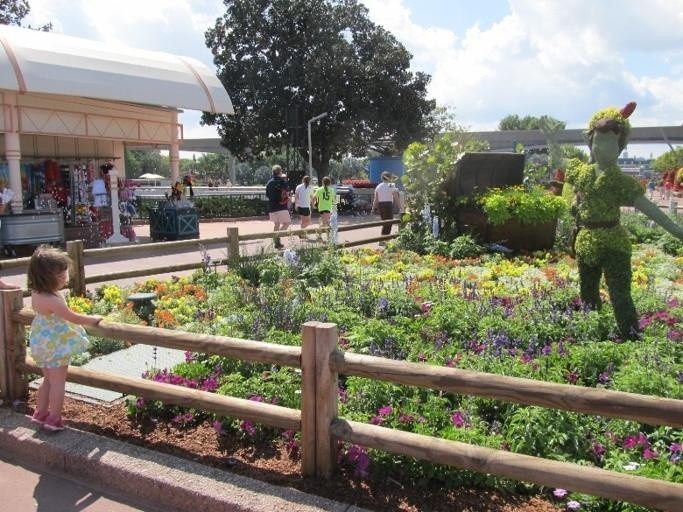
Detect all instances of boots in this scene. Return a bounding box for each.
[272,238,285,249]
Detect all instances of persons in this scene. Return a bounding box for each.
[265,163,292,249]
[537,100,683,341]
[369,171,403,247]
[310,175,336,241]
[0,278,21,290]
[647,176,674,200]
[25,244,103,433]
[293,175,314,240]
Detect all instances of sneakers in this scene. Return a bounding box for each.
[31,409,65,432]
